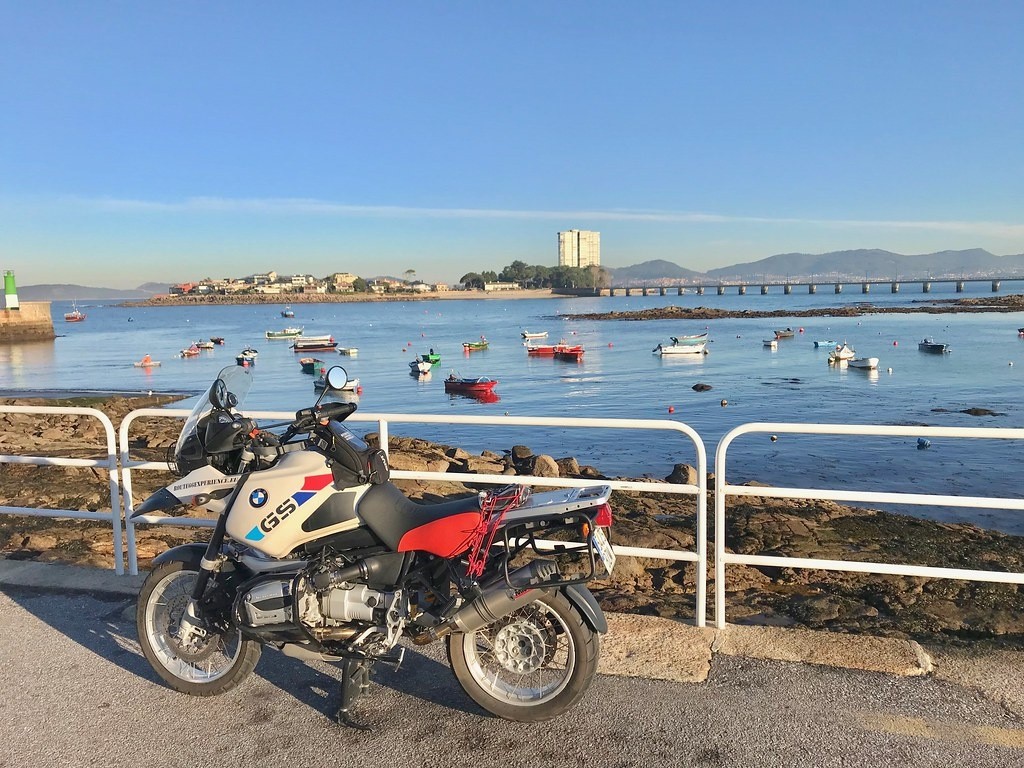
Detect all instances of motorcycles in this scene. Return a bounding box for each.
[130,365,614,730]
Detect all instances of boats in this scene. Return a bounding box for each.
[919,340,951,353]
[660,343,705,354]
[293,334,339,351]
[313,375,359,390]
[134,361,160,366]
[180,349,201,356]
[763,339,778,346]
[301,357,325,370]
[236,347,258,365]
[265,326,304,340]
[1017,327,1024,336]
[848,357,879,368]
[462,336,488,351]
[64,300,86,321]
[522,343,556,354]
[774,329,795,336]
[670,332,708,343]
[521,332,548,338]
[829,339,855,361]
[814,341,837,348]
[339,348,359,355]
[281,301,294,318]
[421,349,442,362]
[409,361,432,372]
[196,342,214,349]
[210,337,224,344]
[444,373,497,391]
[553,338,584,356]
